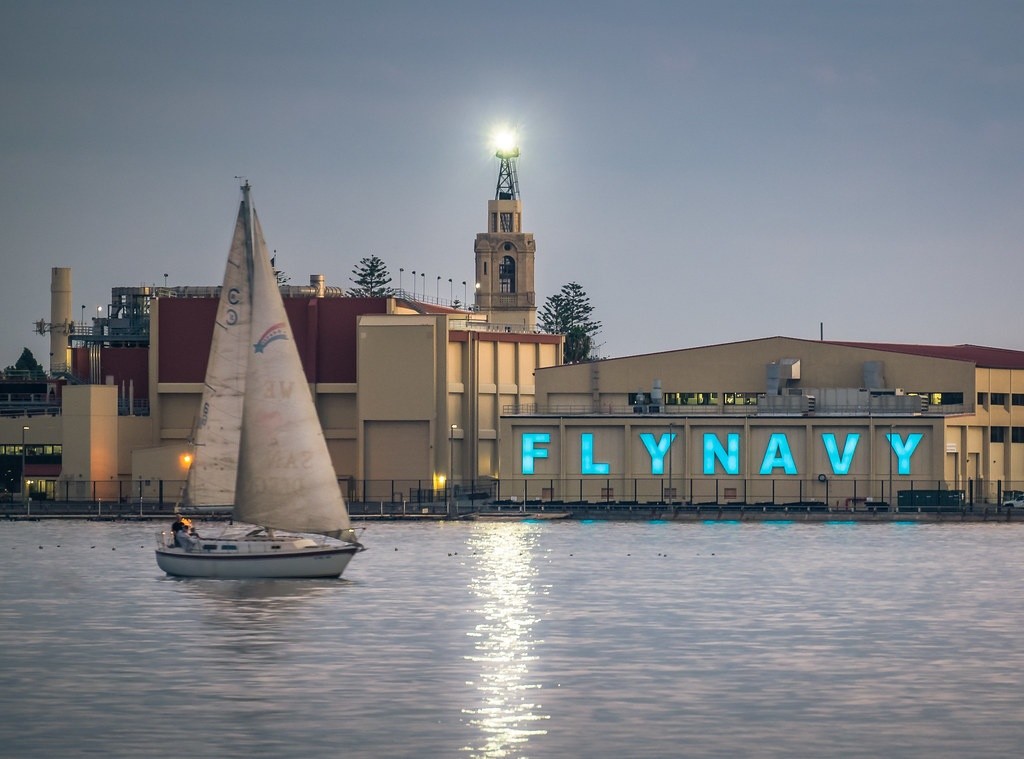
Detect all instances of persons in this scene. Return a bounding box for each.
[172,515,199,548]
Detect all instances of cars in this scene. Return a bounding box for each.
[1003,494,1024,509]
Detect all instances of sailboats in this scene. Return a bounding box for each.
[149,176,372,581]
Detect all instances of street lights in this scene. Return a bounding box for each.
[20,425,31,505]
[96,305,103,318]
[411,270,417,302]
[668,421,677,508]
[447,278,453,307]
[399,268,404,298]
[888,423,897,504]
[163,273,169,286]
[449,422,458,503]
[462,281,468,310]
[421,272,426,302]
[474,284,480,312]
[436,275,441,305]
[81,305,86,347]
